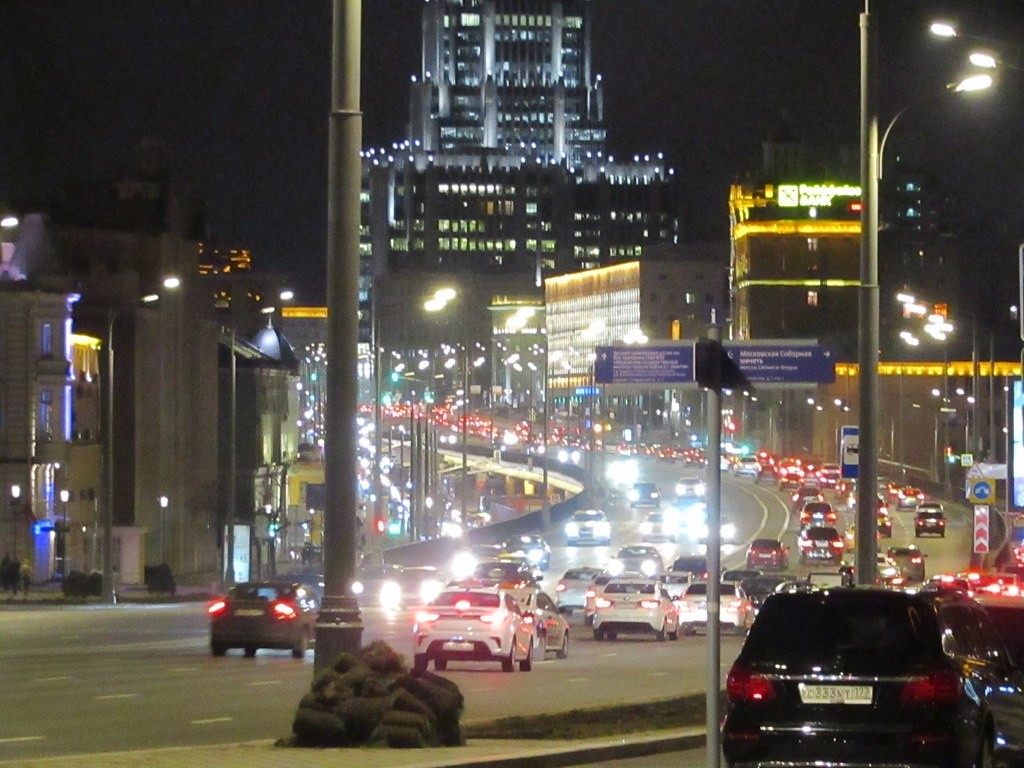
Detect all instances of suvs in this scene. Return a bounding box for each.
[720,572,1024,768]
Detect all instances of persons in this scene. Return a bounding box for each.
[0,553,32,594]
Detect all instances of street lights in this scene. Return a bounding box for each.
[102,294,159,604]
[853,75,996,588]
[505,307,648,524]
[11,485,20,558]
[60,489,69,577]
[896,292,996,494]
[224,303,277,588]
[160,495,168,564]
[309,508,314,558]
[370,289,457,579]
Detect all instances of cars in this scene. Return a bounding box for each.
[212,573,323,658]
[353,400,945,607]
[413,586,531,673]
[506,586,569,660]
[678,580,755,636]
[592,576,679,644]
[555,565,611,613]
